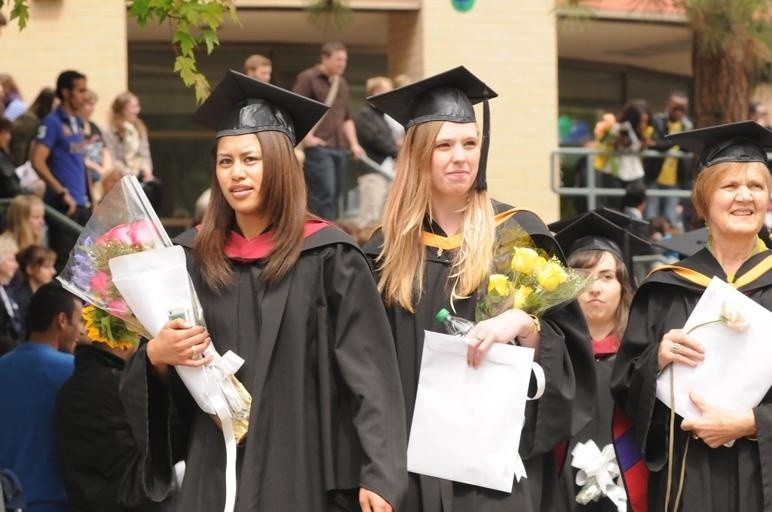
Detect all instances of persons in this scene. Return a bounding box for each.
[611,120,772,512]
[547,207,651,512]
[581,90,704,294]
[356,66,597,512]
[234,40,415,249]
[118,70,407,511]
[3,72,160,511]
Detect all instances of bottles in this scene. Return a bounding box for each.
[435,308,476,338]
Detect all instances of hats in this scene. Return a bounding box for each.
[187,70,330,150]
[548,121,772,291]
[367,66,498,194]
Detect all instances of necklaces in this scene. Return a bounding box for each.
[424,210,463,258]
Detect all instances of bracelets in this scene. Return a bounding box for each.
[517,313,541,339]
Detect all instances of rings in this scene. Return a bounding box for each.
[191,345,196,356]
[672,343,681,353]
[475,335,482,342]
